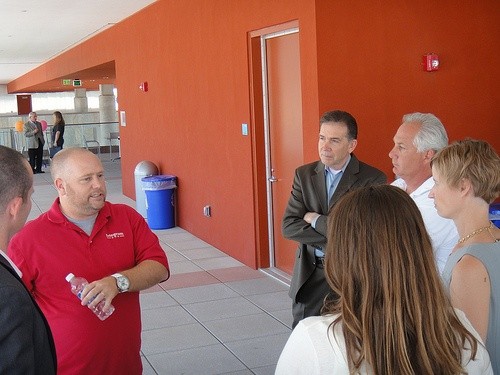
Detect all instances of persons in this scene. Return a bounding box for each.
[274,183,494,375]
[389,112,461,276]
[428,138,500,375]
[23,111,46,174]
[281,110,387,333]
[50,111,65,158]
[0,145,58,375]
[8,147,170,375]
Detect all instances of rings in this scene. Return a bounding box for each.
[100,292,106,298]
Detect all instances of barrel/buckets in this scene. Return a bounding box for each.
[141,175,179,232]
[15,121,24,131]
[40,120,47,131]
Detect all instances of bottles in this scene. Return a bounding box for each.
[65,273,115,321]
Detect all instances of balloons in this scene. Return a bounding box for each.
[40,120,47,131]
[15,121,23,132]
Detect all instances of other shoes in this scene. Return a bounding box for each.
[36,169,44,173]
[33,169,37,174]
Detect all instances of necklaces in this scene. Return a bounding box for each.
[458,221,493,242]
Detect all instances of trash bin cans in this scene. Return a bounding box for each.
[133,160,158,219]
[141,175,177,230]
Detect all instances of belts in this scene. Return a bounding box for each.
[316,258,323,265]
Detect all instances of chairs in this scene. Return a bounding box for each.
[82,135,101,158]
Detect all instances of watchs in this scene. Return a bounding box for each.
[111,273,130,294]
